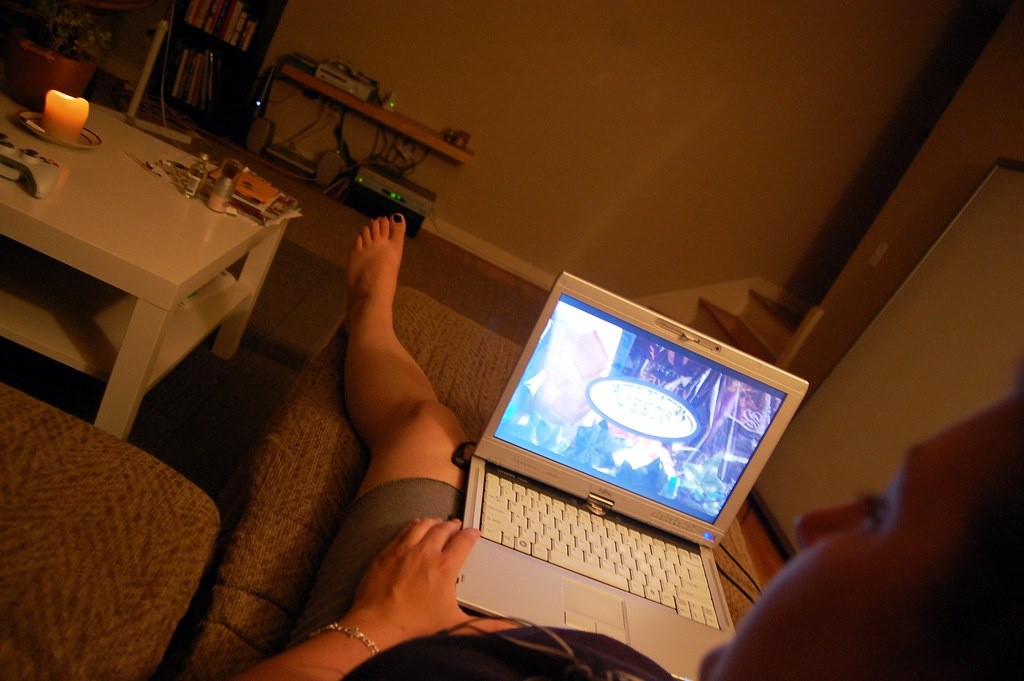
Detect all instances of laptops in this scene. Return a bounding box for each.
[457,271,809,681]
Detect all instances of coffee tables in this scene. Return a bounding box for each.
[0,68,290,441]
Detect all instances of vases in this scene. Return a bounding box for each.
[5,37,98,111]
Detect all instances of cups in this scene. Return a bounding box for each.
[453,130,471,151]
[444,128,457,144]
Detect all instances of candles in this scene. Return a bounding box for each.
[43,89,89,142]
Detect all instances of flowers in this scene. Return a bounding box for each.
[36,0,113,58]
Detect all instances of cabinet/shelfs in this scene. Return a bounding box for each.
[148,0,289,140]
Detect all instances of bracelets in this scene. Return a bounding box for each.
[311,623,379,655]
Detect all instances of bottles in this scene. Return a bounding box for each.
[205,163,240,214]
[181,150,209,201]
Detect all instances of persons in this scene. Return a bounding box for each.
[234,214,1024,681]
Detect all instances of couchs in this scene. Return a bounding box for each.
[178,285,763,681]
[0,383,221,681]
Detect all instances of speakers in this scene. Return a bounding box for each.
[315,151,346,187]
[246,117,275,157]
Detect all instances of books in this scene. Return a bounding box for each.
[208,158,300,228]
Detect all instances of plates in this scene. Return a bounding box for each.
[15,110,103,148]
